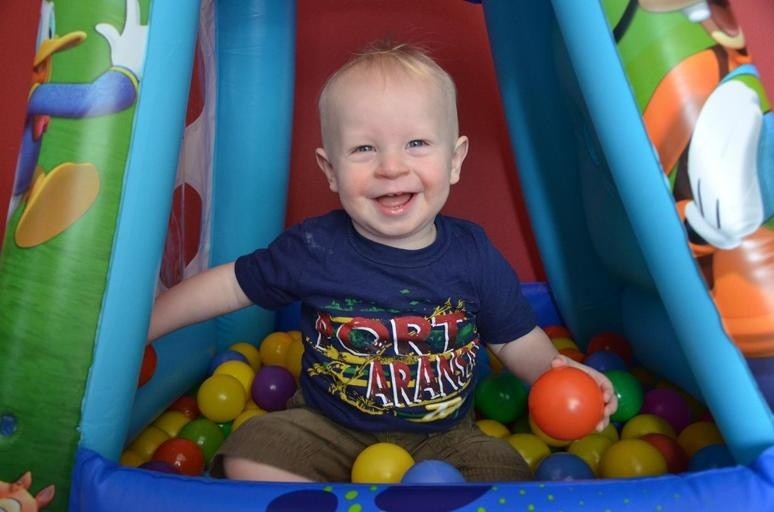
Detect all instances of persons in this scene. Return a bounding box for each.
[148,44,620,482]
